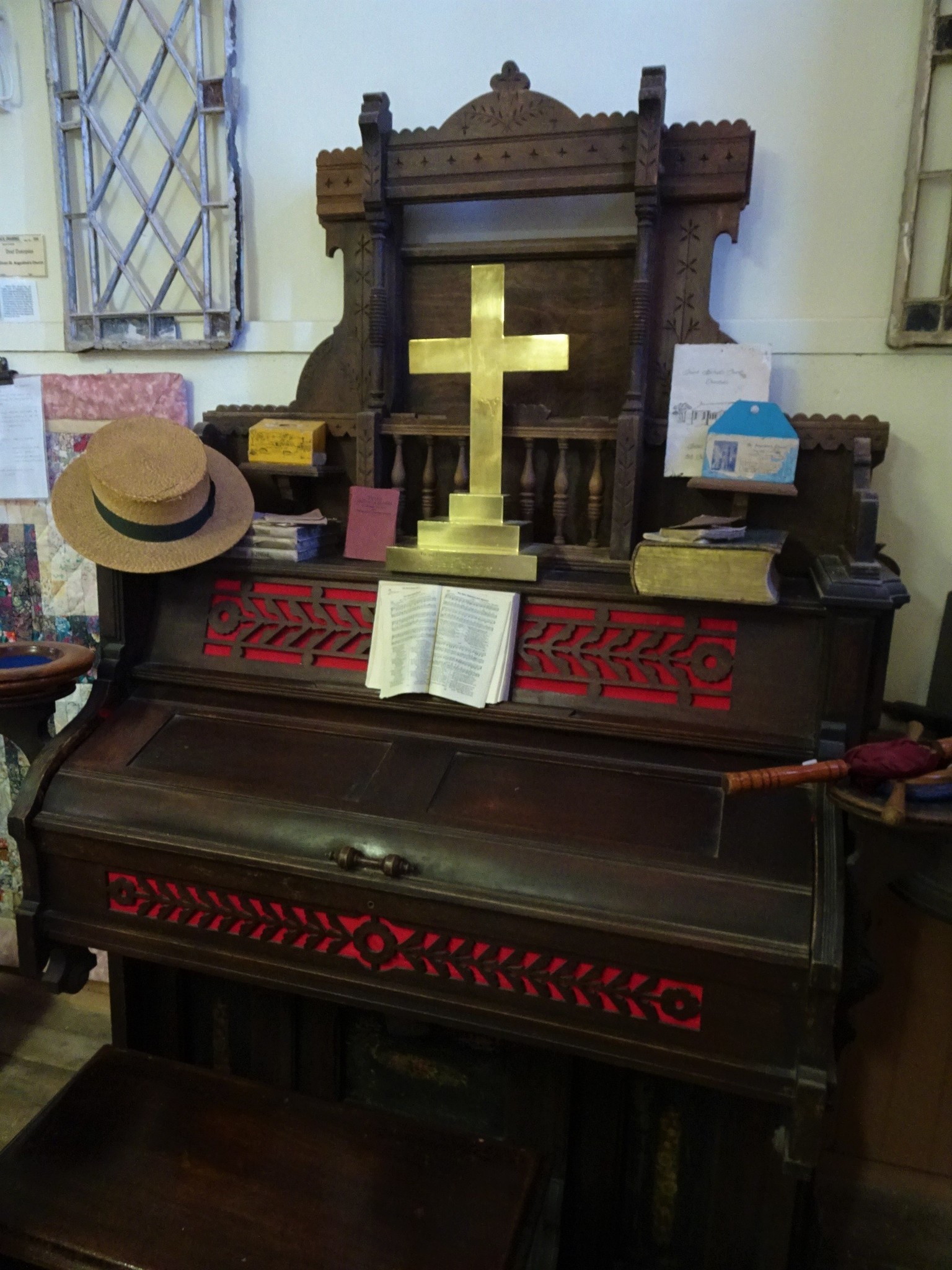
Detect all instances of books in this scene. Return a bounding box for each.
[627,513,792,606]
[216,512,342,563]
[364,580,522,709]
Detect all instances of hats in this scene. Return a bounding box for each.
[47,412,258,574]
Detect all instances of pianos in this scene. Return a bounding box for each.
[6,57,906,1219]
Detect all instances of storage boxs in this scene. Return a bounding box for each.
[248,418,327,465]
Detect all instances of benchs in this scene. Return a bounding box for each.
[0,1044,535,1269]
